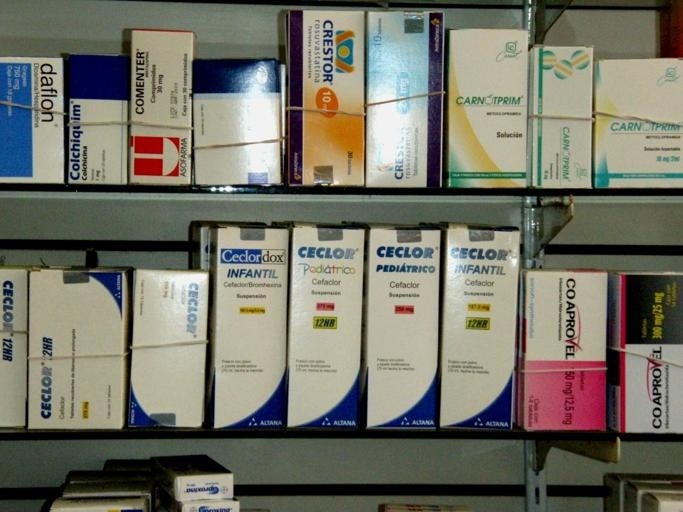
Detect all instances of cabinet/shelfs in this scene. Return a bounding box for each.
[1,1,683,512]
